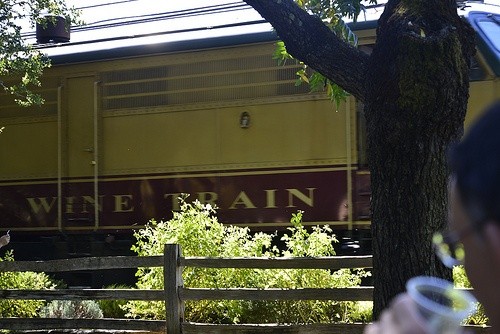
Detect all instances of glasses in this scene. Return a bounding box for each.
[431,214,494,267]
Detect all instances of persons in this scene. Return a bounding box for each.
[364,97,500,334]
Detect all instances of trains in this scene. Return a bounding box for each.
[0,9,500,285]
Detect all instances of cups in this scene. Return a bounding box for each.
[405,276,480,334]
